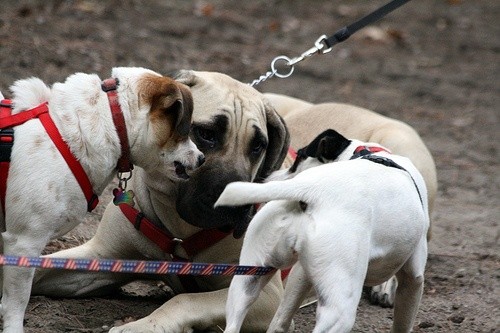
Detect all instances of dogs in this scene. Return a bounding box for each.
[0,65,206,332]
[33,69,438,332]
[213,127,430,333]
